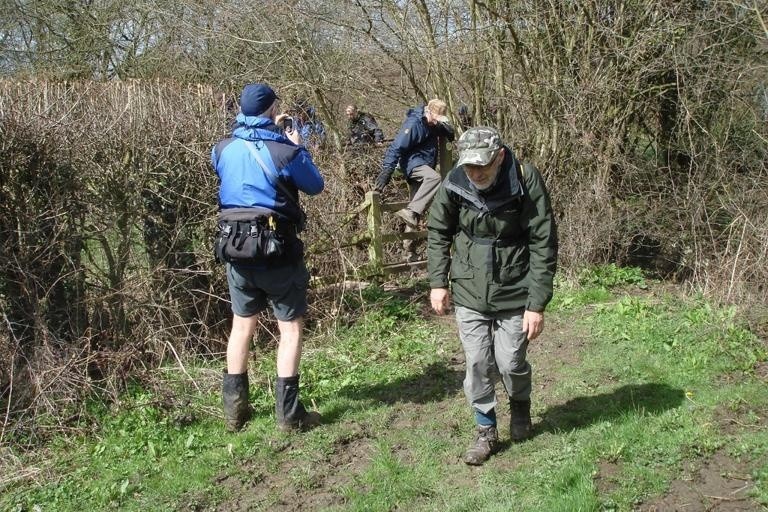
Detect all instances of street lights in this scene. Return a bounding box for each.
[217,207,304,269]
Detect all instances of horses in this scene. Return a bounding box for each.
[284,116,293,135]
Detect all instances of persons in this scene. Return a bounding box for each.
[294,98,497,265]
[425,125,560,464]
[213,82,324,436]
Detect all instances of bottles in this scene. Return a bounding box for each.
[456,126,500,169]
[424,99,450,122]
[240,84,280,116]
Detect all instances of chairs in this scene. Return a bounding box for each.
[509,396,534,442]
[464,424,499,466]
[394,208,418,229]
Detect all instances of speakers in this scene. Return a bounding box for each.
[222,367,252,432]
[275,373,321,432]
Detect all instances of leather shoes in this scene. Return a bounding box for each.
[375,167,393,193]
